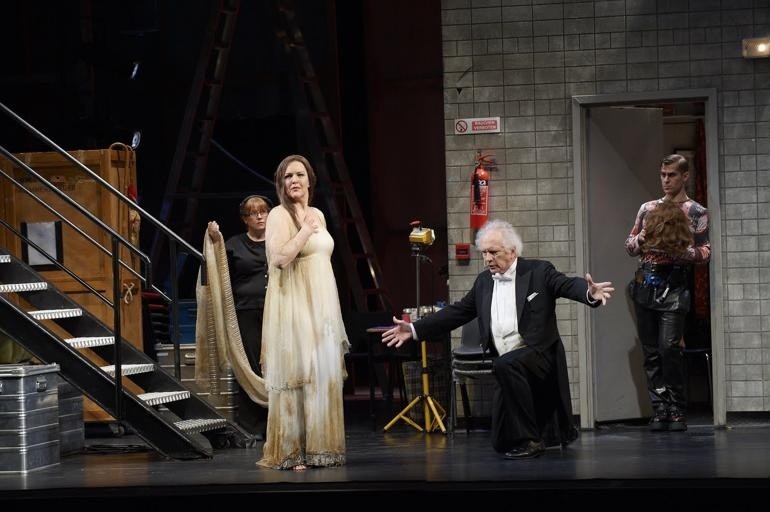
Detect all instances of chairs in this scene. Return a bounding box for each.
[344,310,394,432]
[141,251,197,344]
[679,302,713,418]
[448,317,492,440]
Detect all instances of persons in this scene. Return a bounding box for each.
[623,154,713,432]
[381,220,616,461]
[256,153,353,472]
[206,194,275,441]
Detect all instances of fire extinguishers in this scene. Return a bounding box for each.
[470,154,494,229]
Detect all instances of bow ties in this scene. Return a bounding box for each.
[491,272,512,283]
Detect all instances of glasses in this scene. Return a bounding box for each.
[245,209,268,217]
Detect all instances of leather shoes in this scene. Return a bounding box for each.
[503,437,546,460]
[646,410,688,432]
